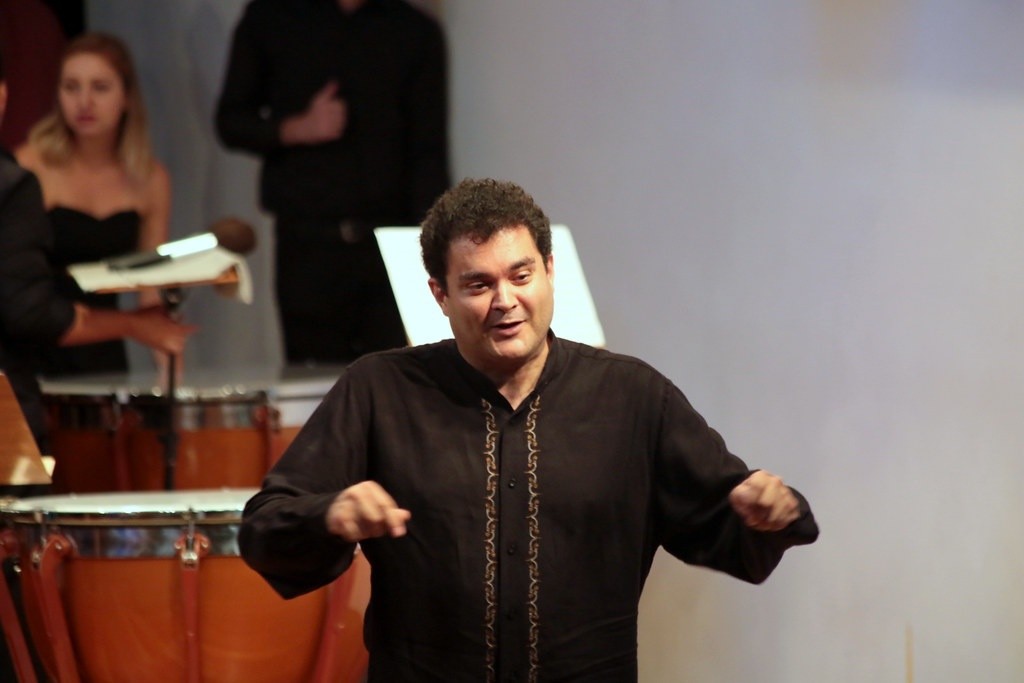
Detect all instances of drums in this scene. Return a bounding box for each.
[0,373,360,682]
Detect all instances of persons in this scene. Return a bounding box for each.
[209,0,454,366]
[237,177,820,683]
[13,32,186,418]
[0,2,203,418]
